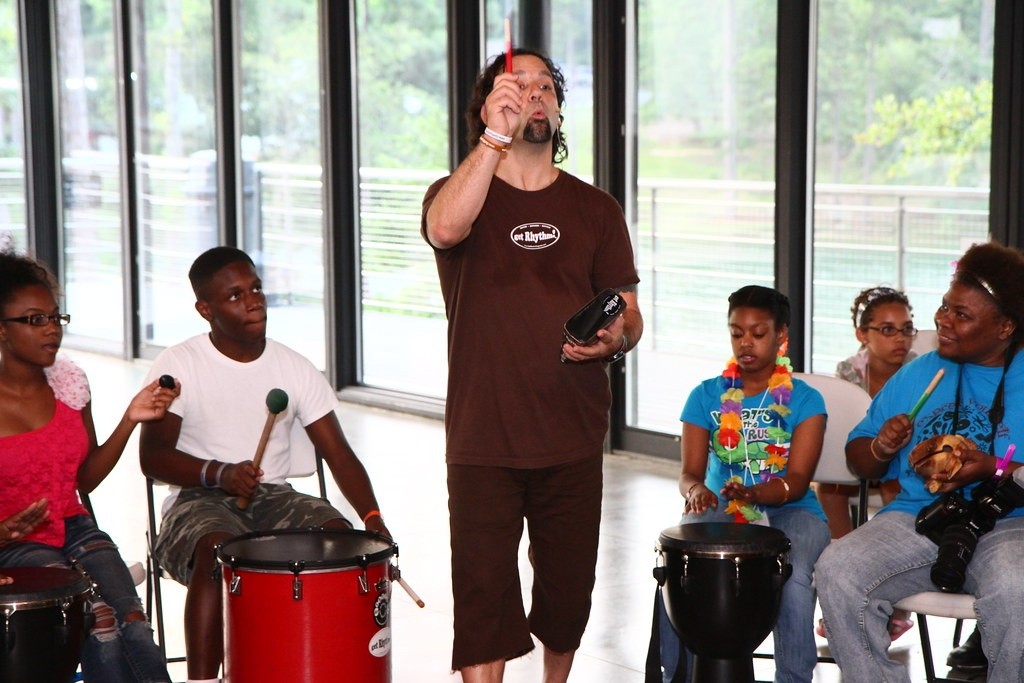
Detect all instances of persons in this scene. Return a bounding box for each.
[140,246,392,683]
[419,48,644,683]
[811,286,921,642]
[0,247,181,683]
[813,243,1024,683]
[661,285,832,683]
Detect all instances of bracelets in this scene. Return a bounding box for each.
[871,437,894,462]
[484,128,512,145]
[216,462,230,487]
[685,482,700,500]
[201,459,216,489]
[362,510,382,525]
[480,135,507,153]
[994,444,1015,480]
[772,475,789,508]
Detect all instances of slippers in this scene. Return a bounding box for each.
[890,618,915,641]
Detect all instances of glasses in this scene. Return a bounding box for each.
[4,314,71,326]
[862,325,919,337]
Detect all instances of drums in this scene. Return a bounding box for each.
[213,527,397,683]
[655,522,795,682]
[0,562,96,683]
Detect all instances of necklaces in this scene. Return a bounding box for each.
[718,336,792,523]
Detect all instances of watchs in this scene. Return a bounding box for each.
[603,334,628,364]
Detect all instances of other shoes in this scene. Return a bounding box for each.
[946,623,988,670]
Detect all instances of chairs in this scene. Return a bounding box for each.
[146,447,327,668]
[754,329,986,683]
